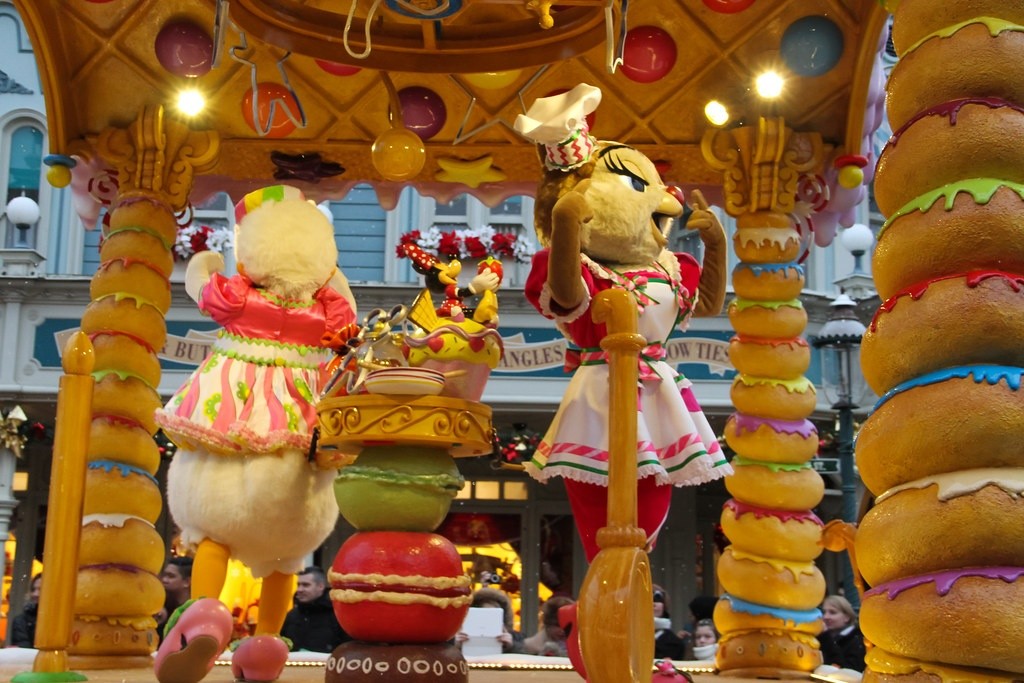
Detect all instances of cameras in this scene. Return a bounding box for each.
[486,574,502,584]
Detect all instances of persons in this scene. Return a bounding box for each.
[281,568,352,655]
[451,590,521,653]
[155,556,194,650]
[10,573,43,648]
[818,594,867,671]
[522,596,577,659]
[650,583,719,662]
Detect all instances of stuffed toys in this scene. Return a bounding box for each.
[513,82,733,680]
[155,185,355,683]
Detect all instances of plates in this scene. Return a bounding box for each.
[365,367,444,395]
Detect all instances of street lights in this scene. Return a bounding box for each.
[810,286,866,609]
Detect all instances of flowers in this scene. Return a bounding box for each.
[174,222,234,263]
[396,224,535,264]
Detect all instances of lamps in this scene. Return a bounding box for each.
[842,224,874,273]
[6,196,40,249]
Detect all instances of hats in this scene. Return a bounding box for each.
[690,597,719,620]
